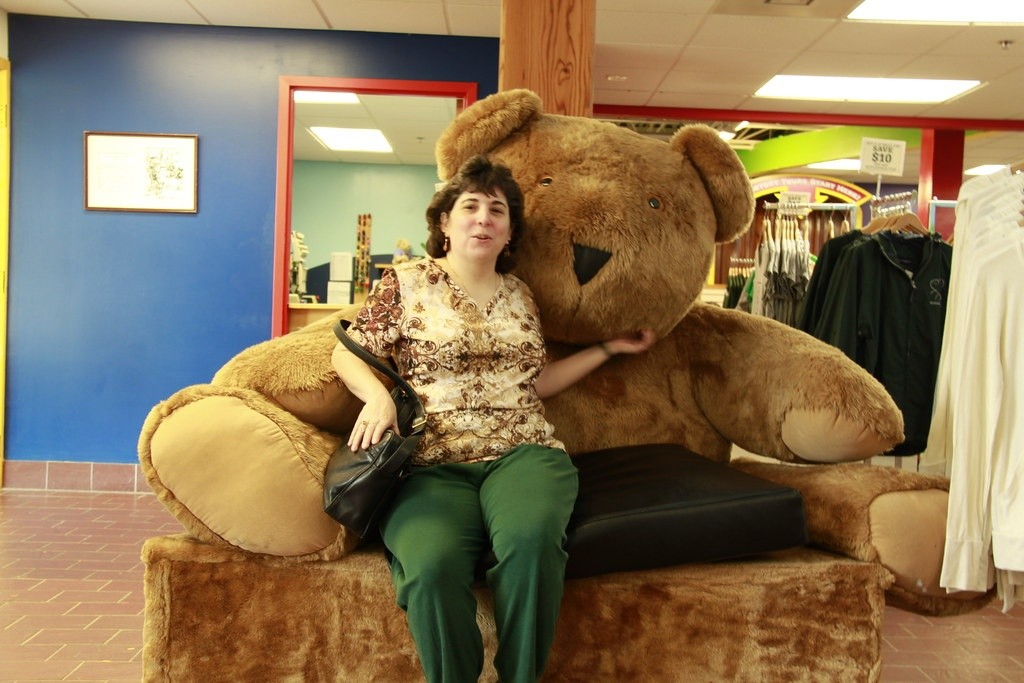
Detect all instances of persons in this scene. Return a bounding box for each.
[328,155,652,683]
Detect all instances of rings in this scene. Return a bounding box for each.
[362,421,367,427]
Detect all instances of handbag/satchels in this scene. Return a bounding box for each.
[322,319,428,539]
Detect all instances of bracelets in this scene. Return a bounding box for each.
[600,342,612,358]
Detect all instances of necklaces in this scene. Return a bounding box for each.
[446,258,500,298]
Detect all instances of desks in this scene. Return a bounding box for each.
[287,303,351,333]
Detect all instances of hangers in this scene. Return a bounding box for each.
[861,192,931,236]
[728,257,755,278]
[757,200,851,246]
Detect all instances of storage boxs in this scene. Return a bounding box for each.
[327,252,353,304]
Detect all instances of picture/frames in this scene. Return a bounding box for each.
[83,132,199,214]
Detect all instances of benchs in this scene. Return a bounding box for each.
[140,531,894,683]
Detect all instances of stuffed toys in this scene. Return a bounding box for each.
[134,87,1000,619]
